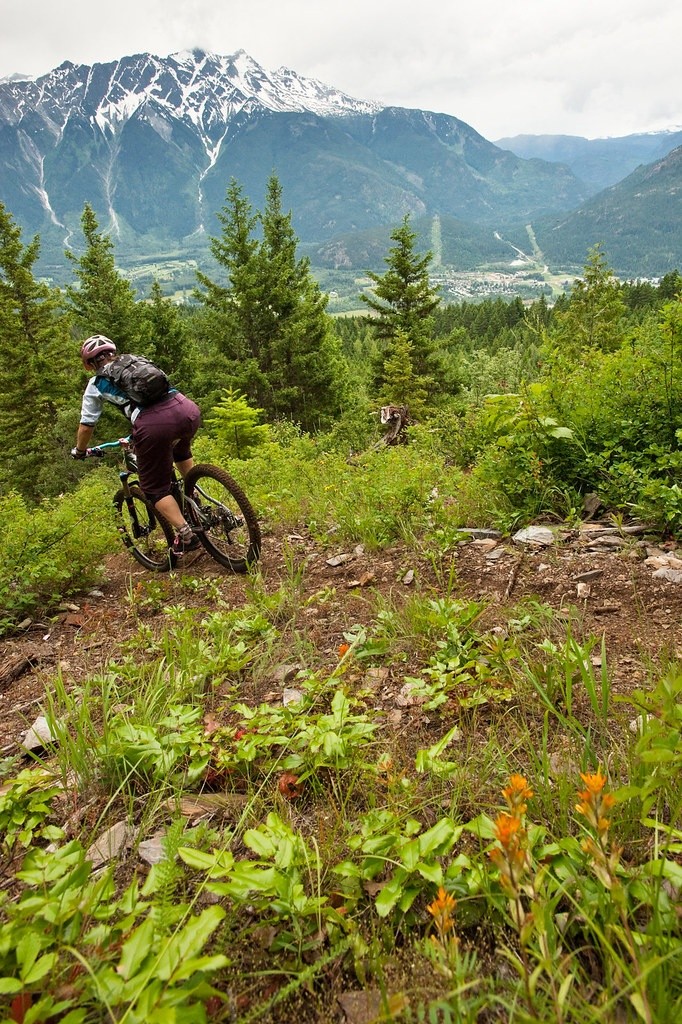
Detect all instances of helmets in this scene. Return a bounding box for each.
[81,335,116,370]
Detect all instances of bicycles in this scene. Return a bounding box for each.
[72,433,262,575]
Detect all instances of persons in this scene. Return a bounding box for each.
[71,335,202,557]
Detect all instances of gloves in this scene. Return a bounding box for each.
[71,447,86,460]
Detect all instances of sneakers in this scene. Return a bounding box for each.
[171,534,201,555]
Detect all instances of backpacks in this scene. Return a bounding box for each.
[95,354,170,406]
